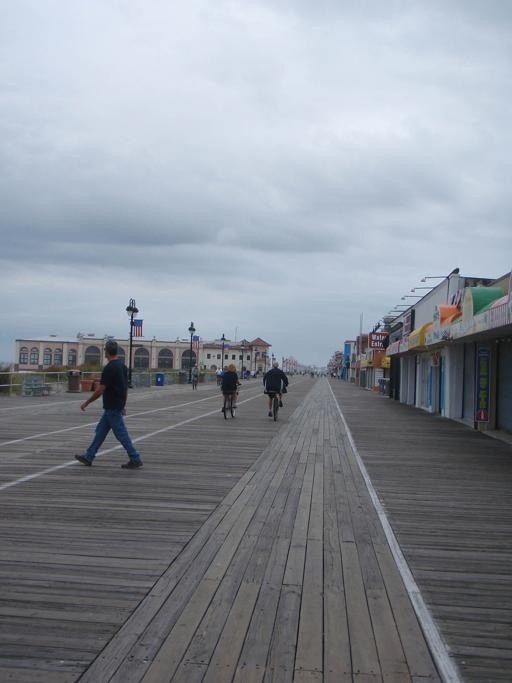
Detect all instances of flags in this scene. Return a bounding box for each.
[224,340,231,353]
[133,320,142,336]
[192,336,199,349]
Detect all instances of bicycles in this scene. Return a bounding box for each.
[268,393,282,421]
[220,382,241,420]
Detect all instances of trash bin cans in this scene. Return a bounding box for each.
[179,371,188,384]
[155,373,164,386]
[66,370,83,393]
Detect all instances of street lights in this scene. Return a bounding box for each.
[125,297,140,387]
[188,321,196,383]
[241,344,244,379]
[220,333,226,371]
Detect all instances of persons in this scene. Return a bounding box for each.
[222,364,241,412]
[216,367,222,386]
[221,366,227,384]
[192,364,198,389]
[263,362,288,417]
[331,372,340,379]
[75,340,143,469]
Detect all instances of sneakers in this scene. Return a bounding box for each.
[75,454,91,465]
[121,460,144,469]
